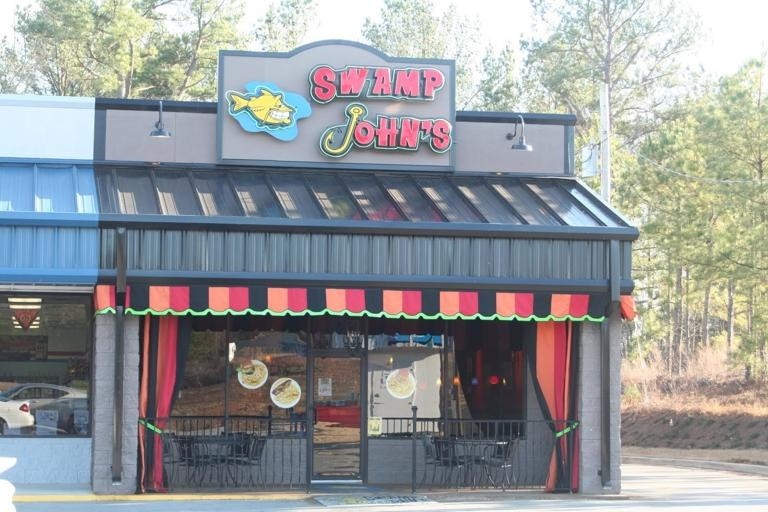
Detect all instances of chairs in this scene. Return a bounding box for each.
[159,431,267,488]
[418,436,517,490]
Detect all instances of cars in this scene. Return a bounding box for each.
[0,383,90,435]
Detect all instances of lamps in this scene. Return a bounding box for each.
[149,101,170,140]
[507,115,532,153]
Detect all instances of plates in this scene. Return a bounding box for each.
[238,360,269,389]
[270,377,301,409]
[385,369,417,400]
